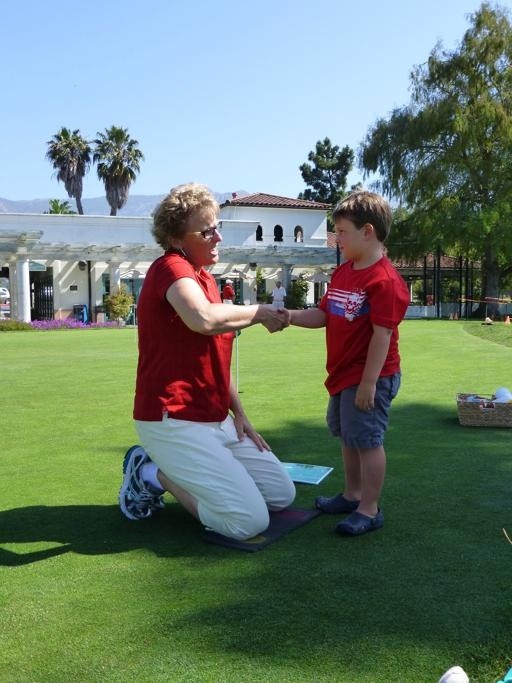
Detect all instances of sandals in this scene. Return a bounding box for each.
[336,505,384,535]
[315,492,361,514]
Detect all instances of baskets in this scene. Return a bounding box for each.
[456,392,512,428]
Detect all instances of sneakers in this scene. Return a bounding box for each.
[151,484,168,513]
[118,444,153,522]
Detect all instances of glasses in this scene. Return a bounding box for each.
[182,219,223,240]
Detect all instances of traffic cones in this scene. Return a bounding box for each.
[452,310,460,320]
[503,314,511,324]
[448,310,455,321]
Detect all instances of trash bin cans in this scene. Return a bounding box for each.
[95,306,106,323]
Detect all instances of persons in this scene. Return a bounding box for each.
[117,182,297,541]
[279,189,409,539]
[269,280,287,308]
[222,279,237,306]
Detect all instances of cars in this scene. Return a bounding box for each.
[0,287,11,304]
